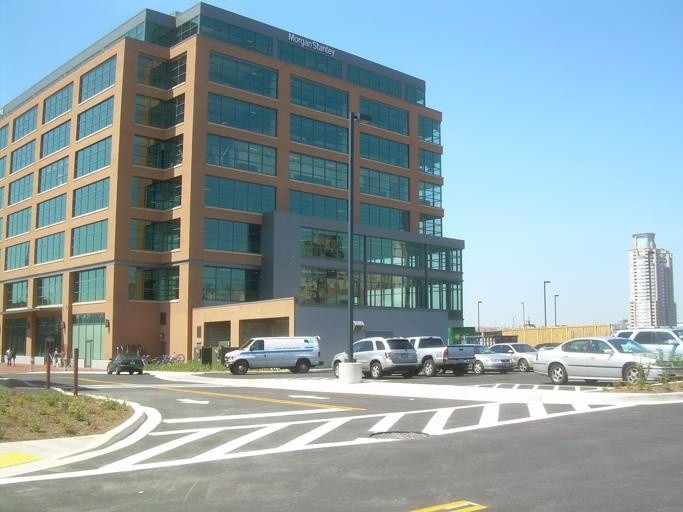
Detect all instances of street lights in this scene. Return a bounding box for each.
[511,281,560,327]
[344,112,372,362]
[477,301,482,332]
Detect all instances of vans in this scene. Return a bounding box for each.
[225,336,321,374]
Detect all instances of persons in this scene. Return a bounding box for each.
[5,345,18,368]
[51,347,71,370]
[28,356,36,372]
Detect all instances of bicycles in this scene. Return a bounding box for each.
[142,353,185,364]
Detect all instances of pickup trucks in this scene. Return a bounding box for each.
[401,336,477,377]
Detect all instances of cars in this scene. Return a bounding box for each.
[468,344,515,374]
[489,342,540,373]
[535,343,562,351]
[107,354,143,375]
[533,336,671,386]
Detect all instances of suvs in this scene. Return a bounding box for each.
[603,328,683,357]
[331,337,419,380]
